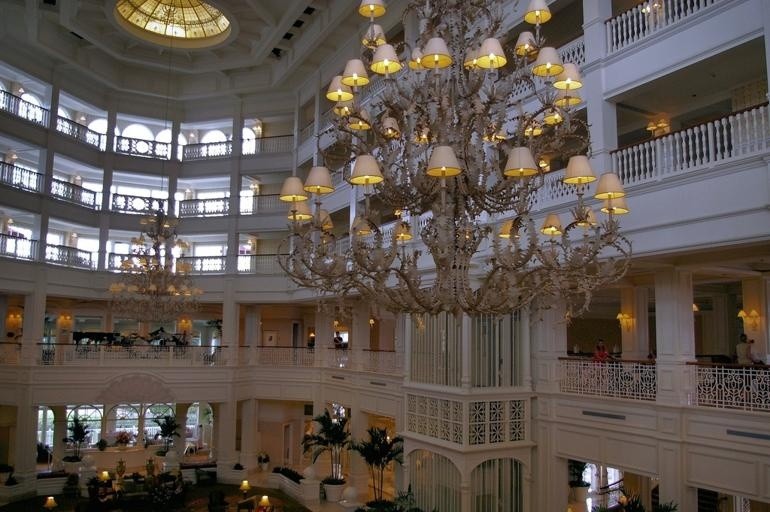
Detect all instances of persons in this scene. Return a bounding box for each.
[592,339,609,362]
[333,331,342,349]
[736,333,755,369]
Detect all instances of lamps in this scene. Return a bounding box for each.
[239,480,251,500]
[616,312,636,332]
[43,496,58,512]
[100,471,111,499]
[275,0,633,328]
[102,0,239,54]
[109,43,203,323]
[646,119,668,137]
[738,309,760,329]
[258,495,272,512]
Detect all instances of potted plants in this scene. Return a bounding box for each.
[301,407,352,502]
[154,415,182,475]
[87,475,105,504]
[256,450,270,472]
[568,459,591,502]
[62,416,90,476]
[352,425,404,512]
[60,473,81,512]
